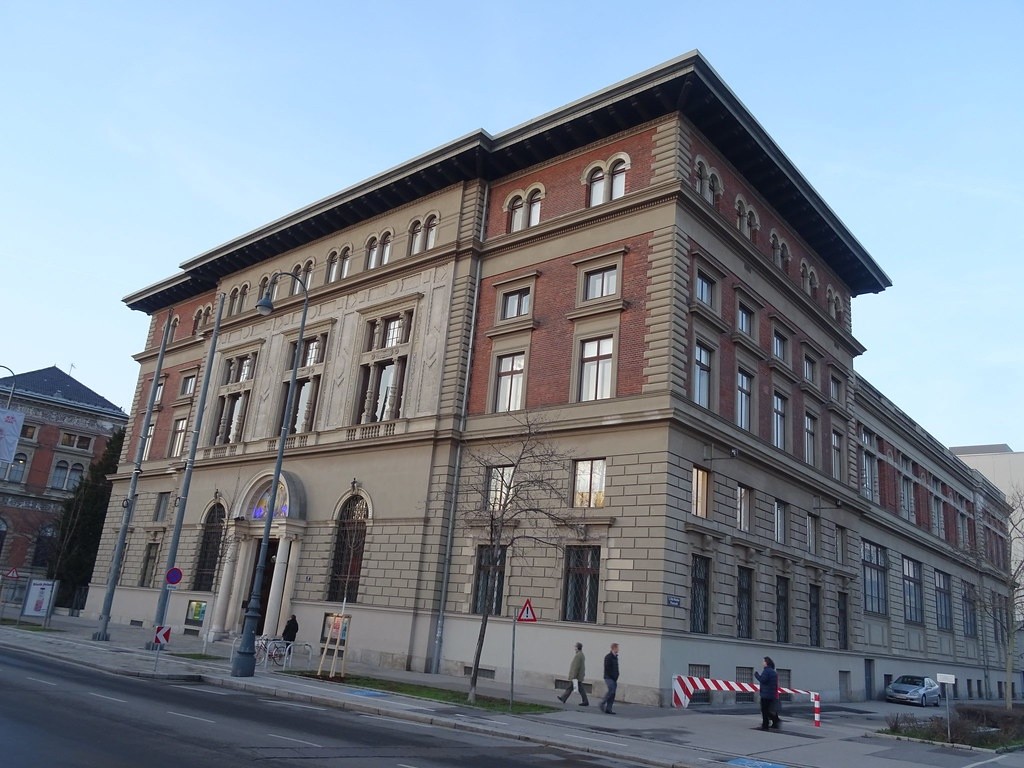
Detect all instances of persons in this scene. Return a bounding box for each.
[557,642,588,706]
[281,614,298,657]
[755,657,781,730]
[598,643,619,715]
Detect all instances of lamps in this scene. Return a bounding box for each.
[704,449,738,461]
[813,500,843,509]
[213,489,220,504]
[350,477,360,495]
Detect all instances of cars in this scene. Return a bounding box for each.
[885,675,942,708]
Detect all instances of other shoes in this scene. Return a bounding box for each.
[771,719,780,728]
[600,703,604,711]
[756,725,769,730]
[557,696,565,704]
[578,702,588,707]
[605,709,615,715]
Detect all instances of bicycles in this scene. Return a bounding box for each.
[254,633,288,665]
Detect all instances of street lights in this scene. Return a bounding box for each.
[229,273,310,680]
